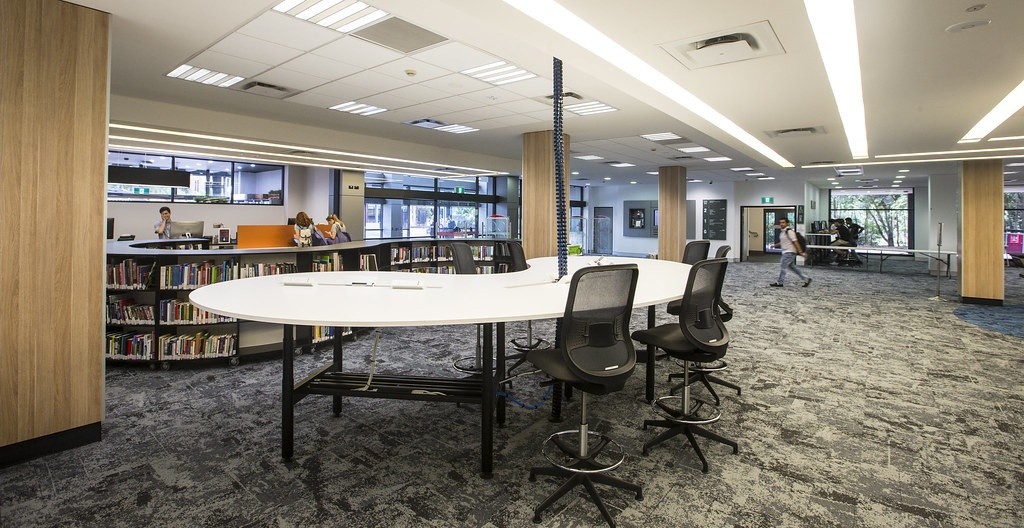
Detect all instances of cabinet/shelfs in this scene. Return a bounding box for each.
[106,238,522,370]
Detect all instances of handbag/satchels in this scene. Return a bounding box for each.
[327,224,352,245]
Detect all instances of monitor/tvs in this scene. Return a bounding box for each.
[814,221,820,231]
[821,221,826,230]
[169,220,204,239]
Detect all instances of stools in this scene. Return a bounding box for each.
[837,237,863,266]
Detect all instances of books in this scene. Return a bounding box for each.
[144,244,202,250]
[390,243,512,274]
[312,252,379,272]
[312,326,352,343]
[240,260,303,279]
[106,255,238,359]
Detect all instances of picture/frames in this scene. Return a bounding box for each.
[811,201,816,209]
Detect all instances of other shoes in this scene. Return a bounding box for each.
[770,283,783,287]
[802,278,812,287]
[829,261,839,265]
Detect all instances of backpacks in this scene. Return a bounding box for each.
[785,228,806,255]
[312,227,327,246]
[297,224,312,247]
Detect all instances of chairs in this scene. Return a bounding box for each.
[450,241,741,528]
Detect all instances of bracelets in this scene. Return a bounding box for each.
[798,251,803,254]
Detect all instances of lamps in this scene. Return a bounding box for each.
[108,150,190,188]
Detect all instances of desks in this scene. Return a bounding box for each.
[189,255,710,479]
[878,248,958,279]
[806,230,838,262]
[805,245,876,269]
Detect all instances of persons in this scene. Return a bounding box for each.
[154,206,172,239]
[769,217,812,287]
[828,216,864,262]
[287,211,352,248]
[427,215,456,238]
